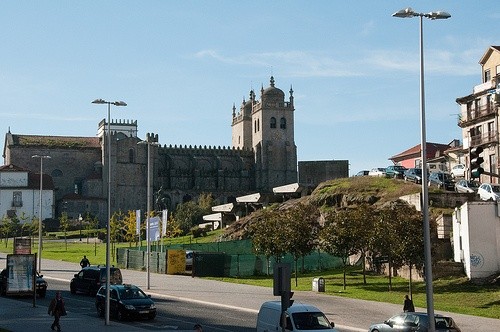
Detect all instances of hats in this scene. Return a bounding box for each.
[56,290,62,294]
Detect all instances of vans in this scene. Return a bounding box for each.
[69,265,122,296]
[256,301,339,332]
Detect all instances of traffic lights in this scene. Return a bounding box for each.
[469,147,484,178]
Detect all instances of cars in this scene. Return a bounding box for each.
[96,284,156,322]
[0,267,48,298]
[451,163,464,178]
[355,170,369,176]
[386,165,406,179]
[369,168,387,177]
[428,171,454,190]
[368,312,462,332]
[477,183,500,202]
[455,180,481,194]
[403,168,422,184]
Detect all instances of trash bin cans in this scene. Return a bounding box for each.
[312,277,325,292]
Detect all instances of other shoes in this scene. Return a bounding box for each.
[50,326,55,330]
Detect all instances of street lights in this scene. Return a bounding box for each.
[136,140,160,290]
[392,8,452,332]
[32,155,52,273]
[92,98,128,325]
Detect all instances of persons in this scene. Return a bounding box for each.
[47,291,67,332]
[192,322,204,332]
[402,294,415,313]
[80,255,90,271]
